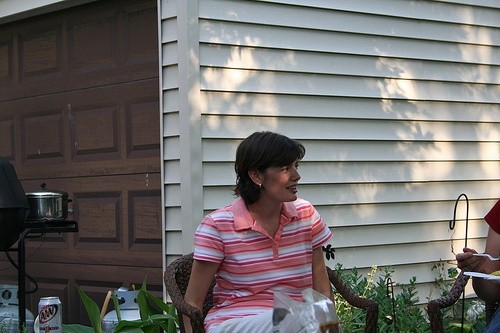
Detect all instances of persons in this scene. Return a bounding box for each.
[456,197,499,333]
[182,131,341,333]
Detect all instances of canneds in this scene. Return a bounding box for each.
[38,296,62,333]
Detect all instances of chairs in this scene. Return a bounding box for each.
[164,253,379,333]
[427,272,500,333]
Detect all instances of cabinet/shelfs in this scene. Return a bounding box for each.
[1,220,79,333]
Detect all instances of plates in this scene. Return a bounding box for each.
[463,271,500,281]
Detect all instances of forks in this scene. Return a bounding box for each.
[472,254,500,260]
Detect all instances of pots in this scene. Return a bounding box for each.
[23,182,74,222]
